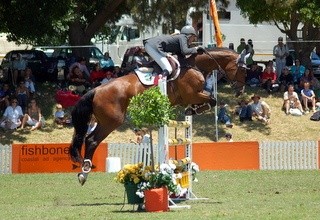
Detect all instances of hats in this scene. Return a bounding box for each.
[251,94,260,99]
[278,38,283,42]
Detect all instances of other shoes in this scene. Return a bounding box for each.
[226,123,233,128]
[305,108,309,113]
[313,108,315,112]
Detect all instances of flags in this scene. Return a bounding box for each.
[210,0,221,46]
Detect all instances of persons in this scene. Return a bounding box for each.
[0,52,41,132]
[218,100,232,127]
[273,37,289,82]
[234,99,252,122]
[53,104,73,126]
[67,52,124,95]
[229,43,234,51]
[129,127,151,166]
[292,57,305,83]
[279,68,293,93]
[143,25,203,80]
[251,95,270,122]
[247,60,281,94]
[284,85,304,114]
[301,82,316,113]
[237,38,249,54]
[310,97,320,121]
[299,69,320,100]
[246,39,254,64]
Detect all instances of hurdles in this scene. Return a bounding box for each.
[147,65,217,210]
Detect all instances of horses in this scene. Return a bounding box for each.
[68,47,251,186]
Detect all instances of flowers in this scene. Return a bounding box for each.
[116,162,200,198]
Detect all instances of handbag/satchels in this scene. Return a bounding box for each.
[286,55,293,66]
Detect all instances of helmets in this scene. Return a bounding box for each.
[181,25,196,36]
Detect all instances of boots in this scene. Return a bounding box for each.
[161,69,168,76]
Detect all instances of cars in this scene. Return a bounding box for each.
[0,50,59,83]
[270,48,320,75]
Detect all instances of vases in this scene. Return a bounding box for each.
[144,185,168,212]
[125,184,143,204]
[177,172,188,186]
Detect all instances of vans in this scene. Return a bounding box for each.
[31,45,113,81]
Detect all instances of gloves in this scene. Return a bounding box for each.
[196,46,205,52]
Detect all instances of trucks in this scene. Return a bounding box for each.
[90,2,305,69]
